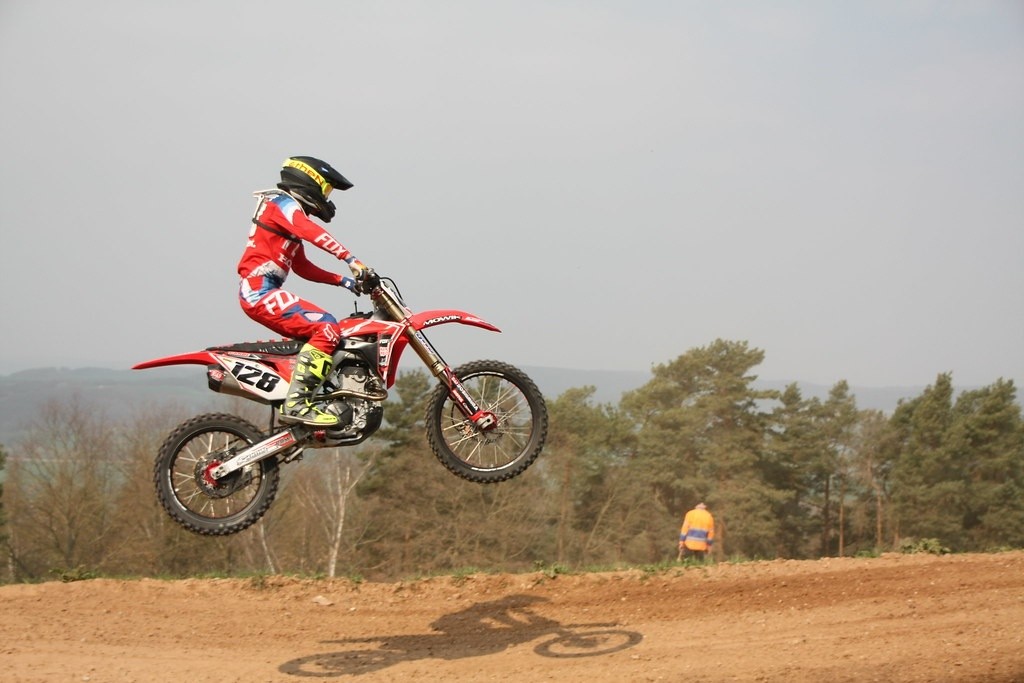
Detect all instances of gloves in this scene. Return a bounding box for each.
[340,277,362,297]
[345,255,369,281]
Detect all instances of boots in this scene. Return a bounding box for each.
[278,342,338,425]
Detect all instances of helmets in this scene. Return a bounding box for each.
[277,156,353,223]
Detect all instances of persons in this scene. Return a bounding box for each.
[678,502,715,566]
[239,157,375,426]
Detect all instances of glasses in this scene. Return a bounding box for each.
[322,183,333,200]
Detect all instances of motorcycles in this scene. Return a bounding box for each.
[129,268,549,536]
